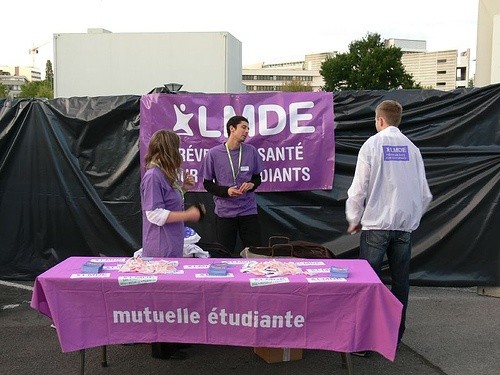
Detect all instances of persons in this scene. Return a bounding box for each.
[203,115,267,256]
[139,129,208,361]
[345,100,432,356]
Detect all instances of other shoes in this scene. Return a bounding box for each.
[153,350,186,359]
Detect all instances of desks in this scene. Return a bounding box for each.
[29,254,405,375]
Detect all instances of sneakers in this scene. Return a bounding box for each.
[352,350,374,357]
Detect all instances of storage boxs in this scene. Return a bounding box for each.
[253,346,303,364]
